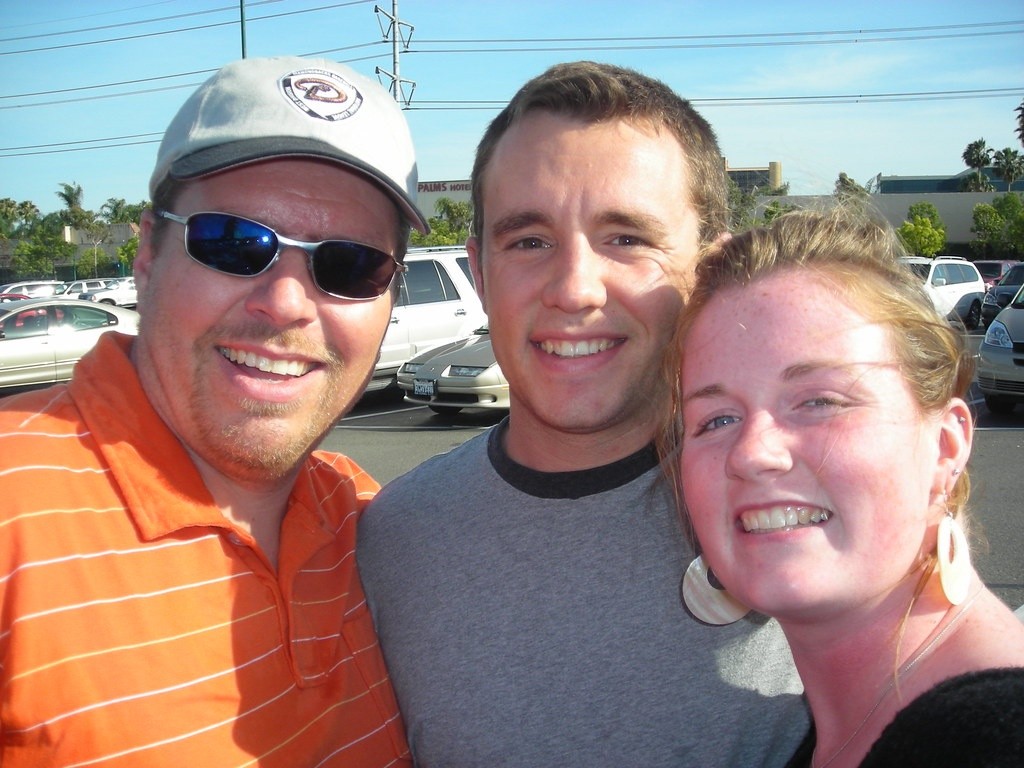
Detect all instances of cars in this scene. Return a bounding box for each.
[396,322,510,417]
[0,298,141,388]
[0,293,65,337]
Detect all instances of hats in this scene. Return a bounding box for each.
[148,56,431,236]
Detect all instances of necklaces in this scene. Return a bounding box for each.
[809,583,987,768]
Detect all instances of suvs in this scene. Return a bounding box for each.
[78,277,138,308]
[0,280,65,301]
[892,255,986,330]
[971,259,1022,294]
[366,245,489,393]
[976,283,1024,416]
[48,278,115,300]
[980,261,1024,331]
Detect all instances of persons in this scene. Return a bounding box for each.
[654,203,1024,768]
[0,55,413,768]
[354,60,813,768]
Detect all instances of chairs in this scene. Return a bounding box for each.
[36,315,48,330]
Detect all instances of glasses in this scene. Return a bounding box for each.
[154,207,409,301]
